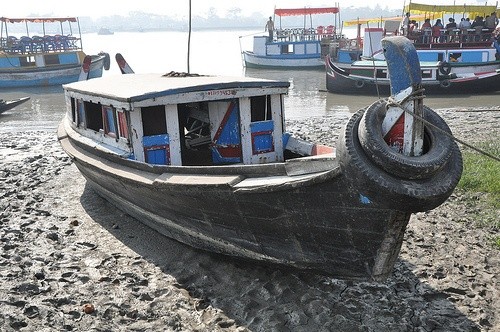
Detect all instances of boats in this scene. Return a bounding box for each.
[324,0,500,98]
[0,95,31,116]
[0,13,111,90]
[54,34,424,286]
[238,1,363,72]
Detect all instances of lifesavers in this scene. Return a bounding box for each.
[103,52,111,71]
[357,95,454,181]
[440,80,451,89]
[439,61,452,76]
[335,102,465,213]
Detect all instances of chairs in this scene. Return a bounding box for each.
[277,25,337,40]
[421,26,431,43]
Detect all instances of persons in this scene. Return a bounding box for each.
[403,12,500,47]
[265,16,274,40]
